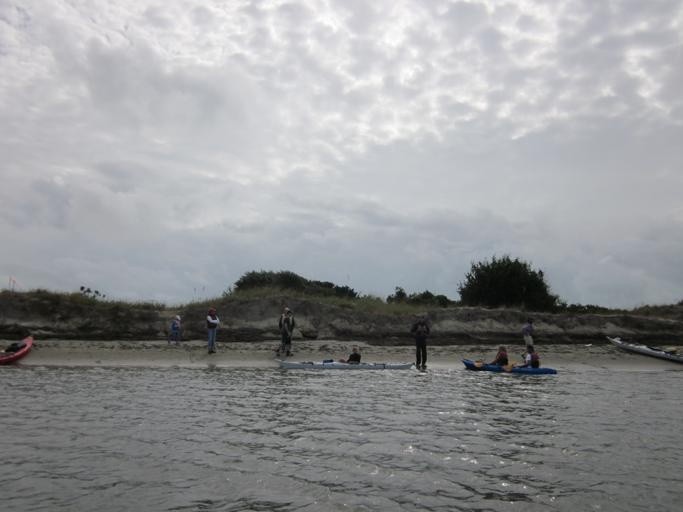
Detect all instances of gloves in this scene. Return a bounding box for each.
[461,357,557,375]
[1,336,34,365]
[607,335,682,363]
[274,356,414,372]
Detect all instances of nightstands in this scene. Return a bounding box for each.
[503,360,518,371]
[474,359,486,368]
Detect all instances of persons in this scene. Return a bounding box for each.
[274,307,296,357]
[482,345,508,366]
[206,308,220,353]
[410,319,430,371]
[167,314,181,345]
[0,345,14,357]
[511,320,539,368]
[339,347,361,365]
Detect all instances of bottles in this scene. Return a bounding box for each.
[208,308,216,313]
[176,315,181,320]
